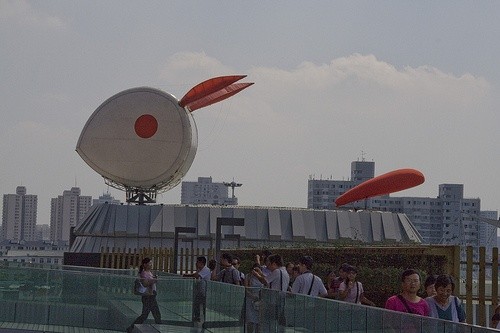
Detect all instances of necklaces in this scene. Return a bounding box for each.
[435,296,445,306]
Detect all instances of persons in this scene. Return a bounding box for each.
[125,257,161,333]
[183,256,211,329]
[210,253,500,333]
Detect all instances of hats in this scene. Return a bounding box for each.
[347,265,357,271]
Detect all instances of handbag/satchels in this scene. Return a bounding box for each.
[133,280,153,297]
